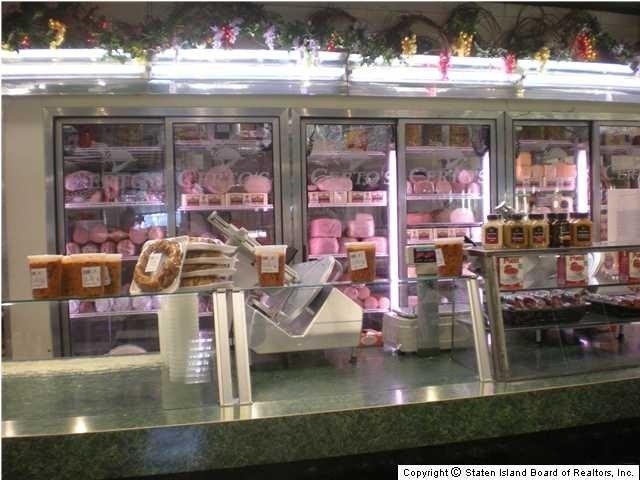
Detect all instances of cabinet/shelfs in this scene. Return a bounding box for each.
[43,103,603,359]
[452,244,640,383]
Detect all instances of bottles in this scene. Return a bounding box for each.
[481,209,595,250]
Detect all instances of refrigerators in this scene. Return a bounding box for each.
[43,106,639,366]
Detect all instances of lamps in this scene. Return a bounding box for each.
[3,48,640,103]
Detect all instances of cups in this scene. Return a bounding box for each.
[27,253,122,300]
[254,234,468,287]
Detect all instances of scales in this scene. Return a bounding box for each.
[383,244,473,358]
